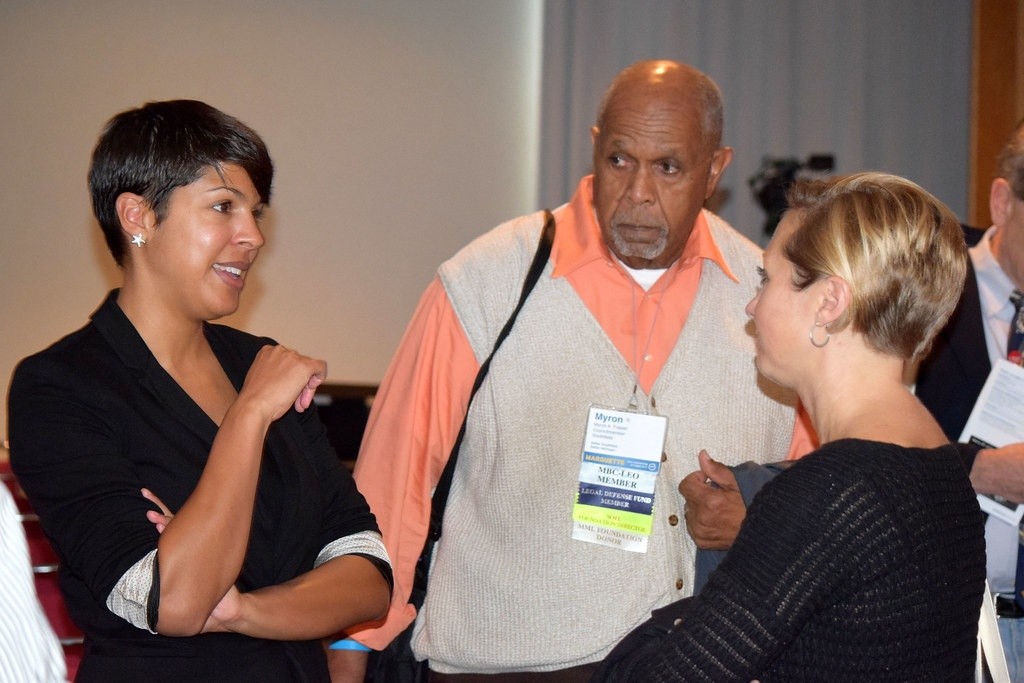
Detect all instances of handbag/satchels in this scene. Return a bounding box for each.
[363,614,430,683]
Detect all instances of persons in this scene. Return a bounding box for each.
[587,172,986,682]
[6,100,395,682]
[317,57,823,682]
[913,113,1022,681]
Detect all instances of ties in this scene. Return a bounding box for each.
[1008,289,1024,369]
